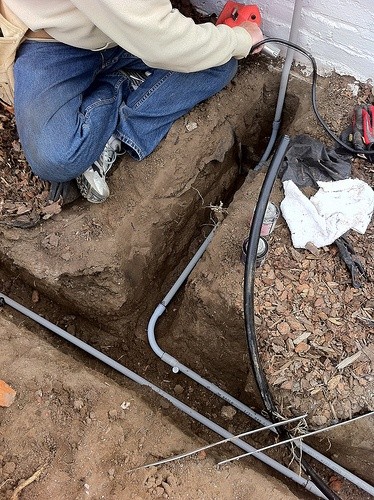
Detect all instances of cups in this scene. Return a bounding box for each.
[250,202,280,238]
[241,236,269,268]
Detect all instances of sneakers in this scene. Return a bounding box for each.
[25,29,54,39]
[76,134,126,205]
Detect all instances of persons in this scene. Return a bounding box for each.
[0,1,265,206]
